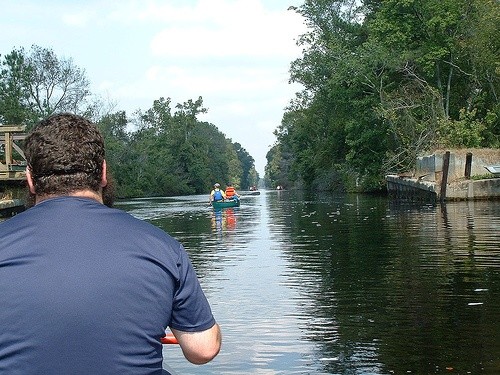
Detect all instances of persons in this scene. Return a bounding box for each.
[0,111,222,375]
[210,183,226,201]
[225,183,240,198]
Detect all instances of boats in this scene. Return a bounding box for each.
[211,201,240,209]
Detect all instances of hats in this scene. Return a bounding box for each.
[214,183,220,187]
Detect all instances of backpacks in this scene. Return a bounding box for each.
[213,189,222,200]
